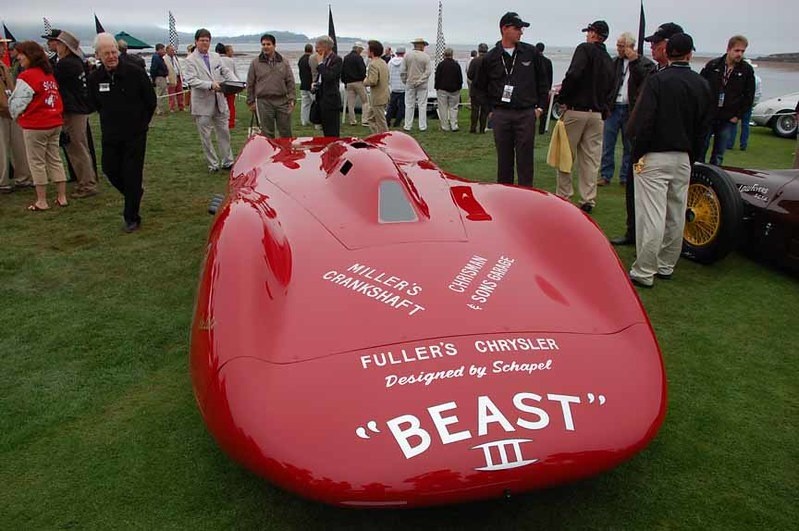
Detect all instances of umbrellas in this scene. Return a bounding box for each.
[110,30,156,52]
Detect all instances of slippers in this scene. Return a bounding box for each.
[52,197,70,207]
[23,200,51,211]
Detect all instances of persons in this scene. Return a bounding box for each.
[88,33,158,233]
[597,30,657,188]
[182,28,240,172]
[465,12,554,188]
[297,35,343,137]
[246,33,297,138]
[399,36,432,131]
[341,40,409,134]
[434,48,464,131]
[699,35,764,166]
[556,20,614,213]
[0,28,101,212]
[150,41,244,131]
[629,23,712,289]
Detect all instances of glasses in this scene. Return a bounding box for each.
[588,23,598,31]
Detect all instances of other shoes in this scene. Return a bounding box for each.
[597,177,610,186]
[350,120,370,127]
[124,223,138,233]
[302,121,322,130]
[610,237,634,245]
[170,108,188,113]
[630,272,675,289]
[0,185,12,193]
[539,127,545,134]
[15,177,36,187]
[208,164,233,174]
[581,202,593,214]
[70,181,98,199]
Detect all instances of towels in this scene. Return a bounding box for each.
[545,118,574,173]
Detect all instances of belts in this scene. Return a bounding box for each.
[567,105,602,112]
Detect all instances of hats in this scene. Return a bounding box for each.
[55,30,84,60]
[743,59,758,68]
[41,29,61,39]
[582,20,609,37]
[643,22,684,43]
[395,47,406,54]
[500,11,530,28]
[353,41,366,50]
[666,33,696,57]
[410,37,430,46]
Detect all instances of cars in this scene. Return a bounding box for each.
[188,129,668,514]
[682,160,799,285]
[547,82,565,121]
[337,65,462,120]
[750,90,799,140]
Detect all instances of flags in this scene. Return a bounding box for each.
[434,0,448,78]
[94,12,107,34]
[637,0,646,56]
[327,2,337,56]
[168,9,181,53]
[2,23,17,44]
[43,16,54,38]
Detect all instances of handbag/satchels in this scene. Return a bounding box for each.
[247,109,262,139]
[309,99,323,125]
